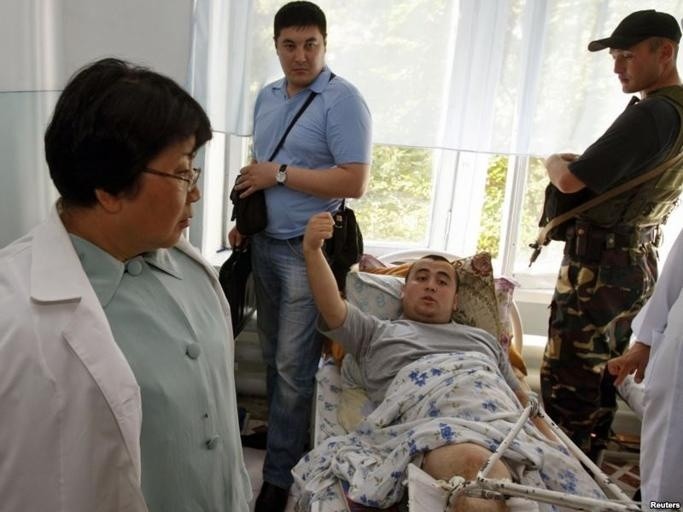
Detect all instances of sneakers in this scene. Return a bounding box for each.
[238,430,268,451]
[253,480,291,512]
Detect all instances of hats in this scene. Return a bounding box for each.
[588,9,682,53]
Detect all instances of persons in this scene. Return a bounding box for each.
[228,1,372,512]
[540,10,681,460]
[0,59,257,512]
[605,230,683,510]
[303,211,560,510]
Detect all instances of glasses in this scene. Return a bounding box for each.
[141,166,202,186]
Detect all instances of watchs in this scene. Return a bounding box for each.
[276,162,288,184]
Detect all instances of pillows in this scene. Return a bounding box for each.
[340,251,520,388]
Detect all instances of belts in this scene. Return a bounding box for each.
[582,227,663,251]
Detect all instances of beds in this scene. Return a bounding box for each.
[289,249,616,512]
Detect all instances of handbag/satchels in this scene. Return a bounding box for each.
[218,236,258,340]
[230,174,269,236]
[527,181,581,267]
[323,202,368,293]
